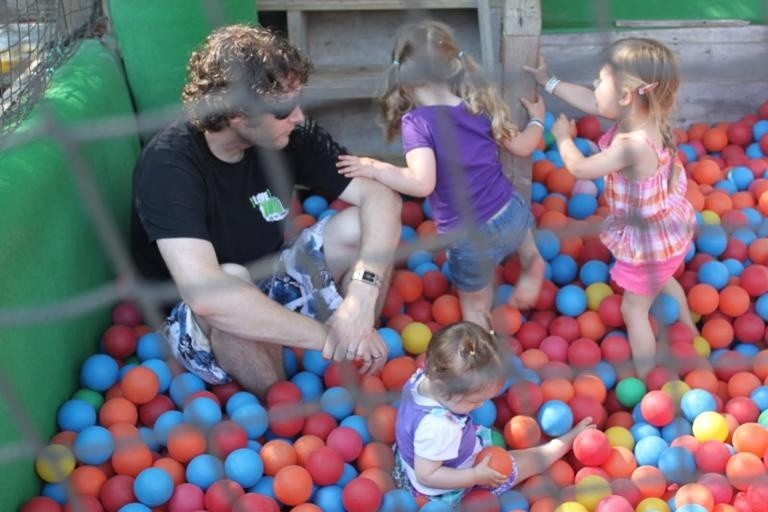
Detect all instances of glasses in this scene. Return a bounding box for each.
[254,87,300,120]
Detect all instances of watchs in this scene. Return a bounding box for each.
[350,270,383,286]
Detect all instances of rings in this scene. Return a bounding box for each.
[372,353,383,359]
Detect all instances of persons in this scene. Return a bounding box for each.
[526,39,700,378]
[335,20,545,333]
[131,22,403,406]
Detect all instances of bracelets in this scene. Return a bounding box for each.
[543,75,560,95]
[528,116,545,130]
